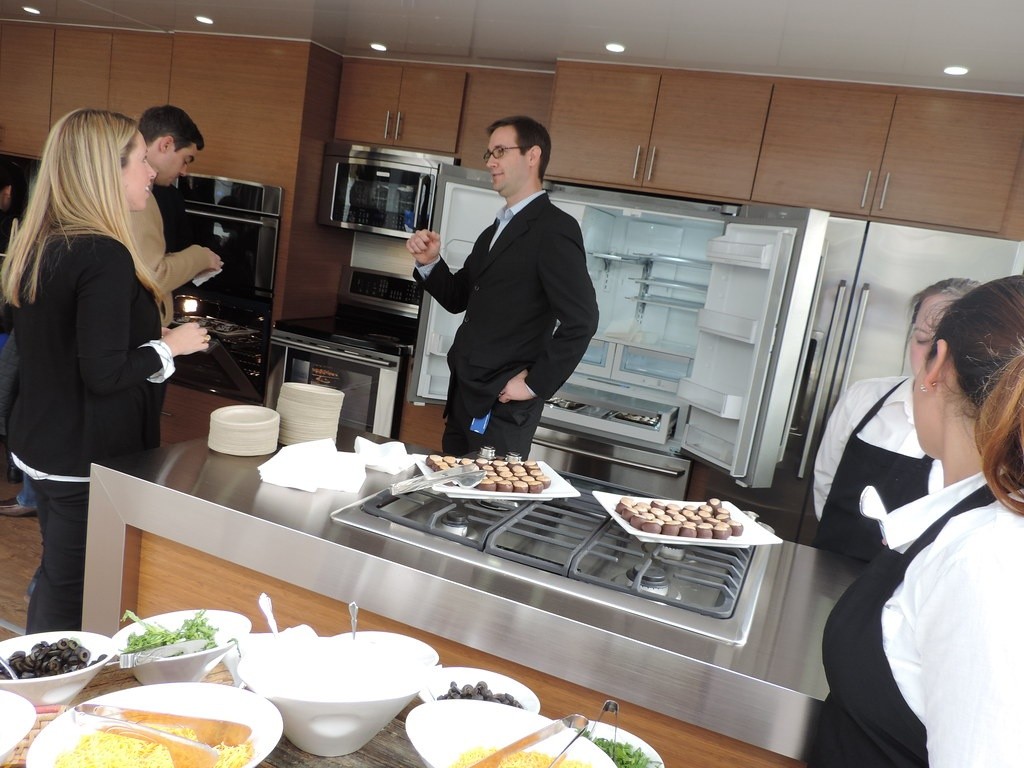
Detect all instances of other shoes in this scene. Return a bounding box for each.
[7,467,23,484]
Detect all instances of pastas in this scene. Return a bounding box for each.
[52,721,256,768]
[443,747,592,768]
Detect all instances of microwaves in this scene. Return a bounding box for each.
[317,138,460,240]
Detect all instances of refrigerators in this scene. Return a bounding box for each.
[406,159,1024,548]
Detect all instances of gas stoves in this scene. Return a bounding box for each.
[330,444,777,649]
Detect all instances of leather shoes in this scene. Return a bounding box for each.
[0,497,37,517]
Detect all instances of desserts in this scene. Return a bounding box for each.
[426,454,551,494]
[615,496,743,540]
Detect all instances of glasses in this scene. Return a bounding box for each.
[483,145,532,163]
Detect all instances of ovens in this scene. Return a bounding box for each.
[262,338,407,440]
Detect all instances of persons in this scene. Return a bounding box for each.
[807,275,1024,768]
[813,277,984,561]
[407,115,599,462]
[129,104,224,328]
[0,109,212,635]
[0,158,43,518]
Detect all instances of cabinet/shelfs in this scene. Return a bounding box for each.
[0,17,1024,242]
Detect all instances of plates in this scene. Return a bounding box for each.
[591,491,783,548]
[207,382,345,456]
[412,454,582,502]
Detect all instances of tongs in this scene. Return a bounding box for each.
[71,704,252,768]
[390,463,488,496]
[120,637,209,669]
[591,700,618,766]
[468,713,588,767]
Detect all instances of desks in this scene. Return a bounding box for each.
[0,655,423,768]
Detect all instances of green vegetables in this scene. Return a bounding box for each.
[582,730,659,768]
[118,607,243,659]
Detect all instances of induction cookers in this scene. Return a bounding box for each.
[271,265,425,358]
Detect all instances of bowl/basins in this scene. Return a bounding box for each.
[0,631,116,768]
[112,609,252,686]
[26,683,283,768]
[236,630,665,768]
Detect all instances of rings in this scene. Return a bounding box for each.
[205,334,211,343]
